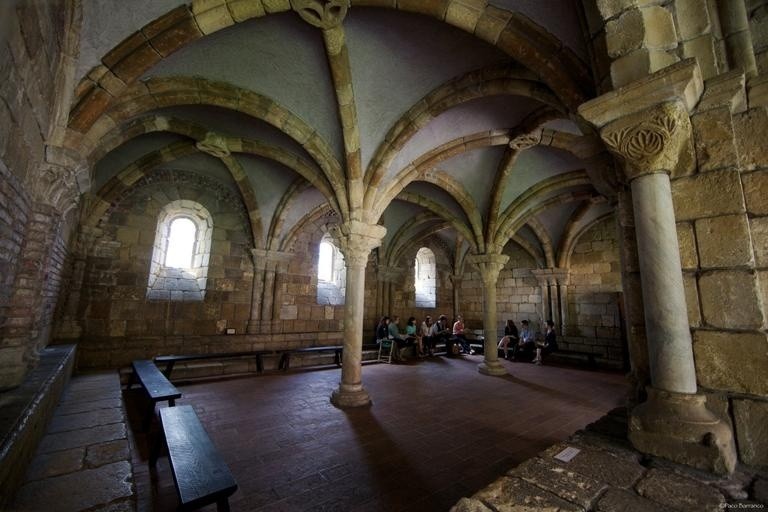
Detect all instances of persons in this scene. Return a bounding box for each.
[496,319,520,360]
[420,315,436,356]
[432,315,457,358]
[375,316,400,360]
[509,320,537,363]
[531,320,557,366]
[387,314,409,362]
[405,316,429,360]
[452,313,476,355]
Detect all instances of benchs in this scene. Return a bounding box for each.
[124,358,183,437]
[361,333,607,372]
[155,347,276,381]
[275,345,343,371]
[160,401,244,511]
